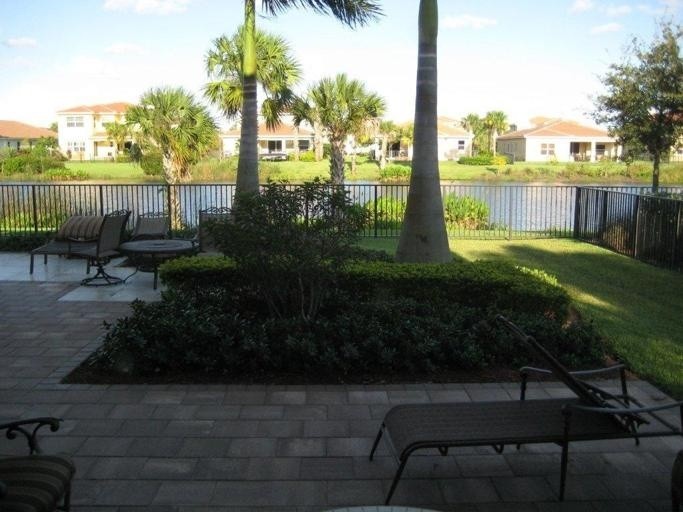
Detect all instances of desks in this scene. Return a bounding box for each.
[117,239,199,290]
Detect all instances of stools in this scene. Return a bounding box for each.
[1,414,76,512]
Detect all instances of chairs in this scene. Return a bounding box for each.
[27,209,170,287]
[368,314,681,503]
[192,206,233,257]
